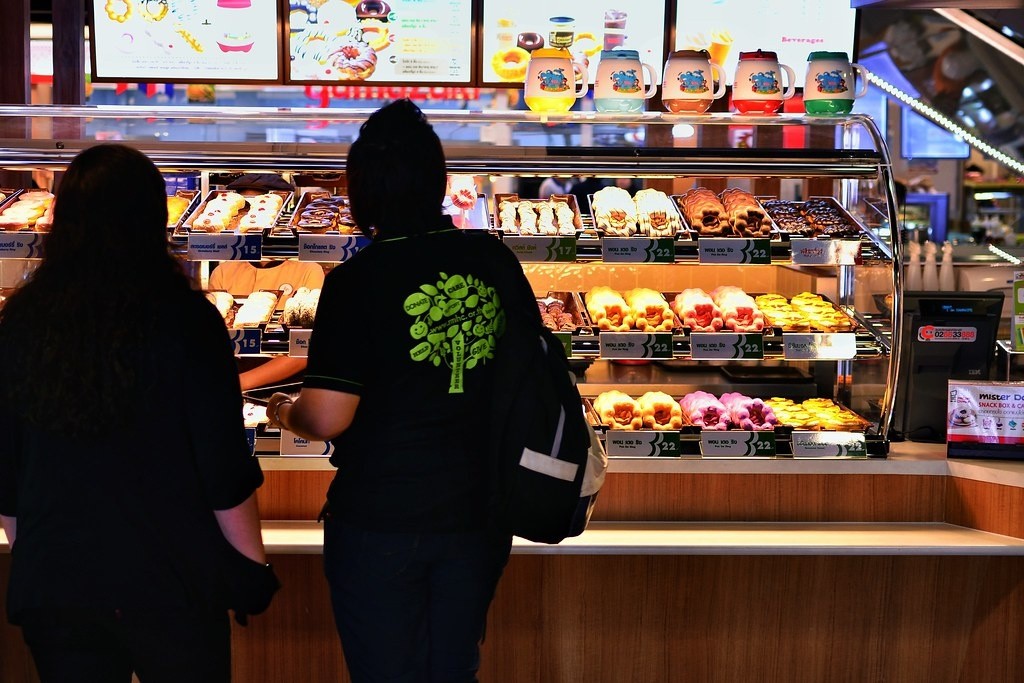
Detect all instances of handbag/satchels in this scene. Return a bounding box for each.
[464,229,607,545]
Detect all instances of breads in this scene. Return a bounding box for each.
[203,287,321,329]
[167,192,284,231]
[499,201,576,235]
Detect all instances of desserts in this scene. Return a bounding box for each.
[755,292,853,333]
[764,397,867,432]
[536,300,577,331]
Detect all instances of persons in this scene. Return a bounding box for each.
[265,100,541,683]
[0,143,280,683]
[963,164,1004,245]
[207,175,327,393]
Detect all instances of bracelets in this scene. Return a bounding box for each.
[274,400,292,429]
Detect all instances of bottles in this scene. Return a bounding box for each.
[922,241,940,291]
[906,241,923,292]
[938,241,957,292]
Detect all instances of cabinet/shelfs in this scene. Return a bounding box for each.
[0,104,906,461]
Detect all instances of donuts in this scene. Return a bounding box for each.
[104,0,169,23]
[585,284,764,333]
[0,190,56,232]
[592,187,856,241]
[492,32,545,80]
[296,197,361,234]
[287,0,393,82]
[592,390,778,431]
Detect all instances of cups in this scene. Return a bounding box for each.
[605,9,629,52]
[661,49,726,114]
[803,51,867,114]
[523,48,589,113]
[592,49,657,113]
[732,48,796,114]
[548,16,575,47]
[214,0,254,53]
